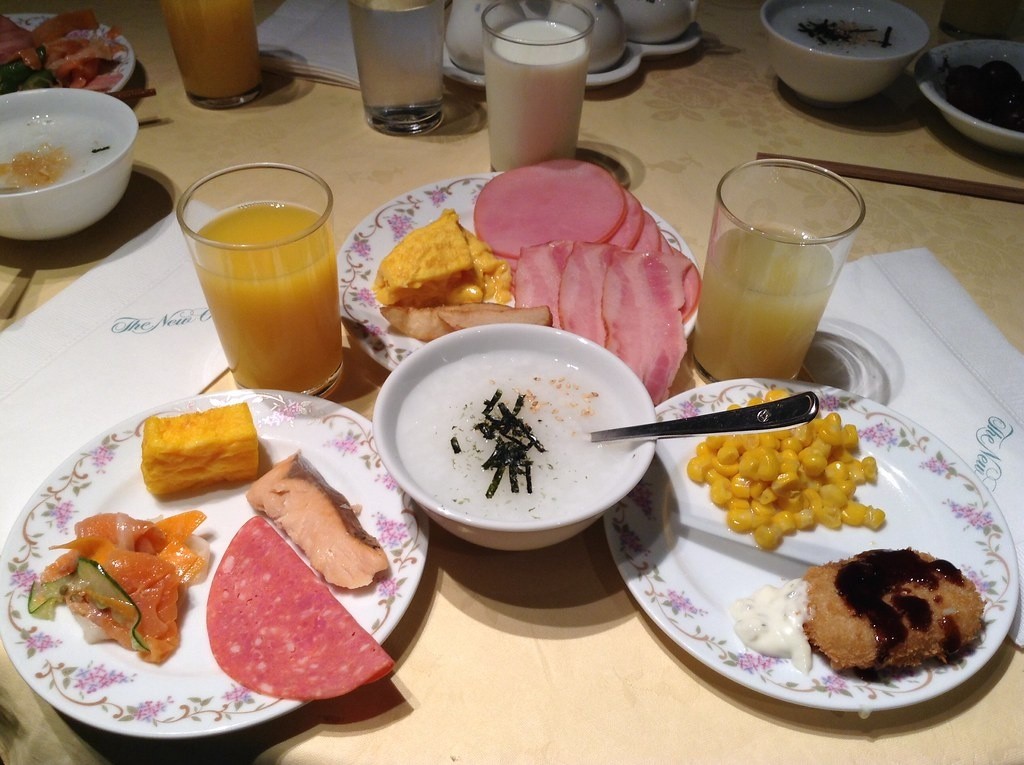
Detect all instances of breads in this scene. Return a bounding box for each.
[140,403,260,494]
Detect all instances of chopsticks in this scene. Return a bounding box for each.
[756,152,1024,204]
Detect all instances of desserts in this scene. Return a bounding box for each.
[732,549,987,674]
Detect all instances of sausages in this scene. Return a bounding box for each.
[206,515,395,698]
[473,160,700,323]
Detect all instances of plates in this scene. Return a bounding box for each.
[335,170,702,371]
[0,12,137,96]
[443,22,702,89]
[603,375,1020,712]
[0,389,430,738]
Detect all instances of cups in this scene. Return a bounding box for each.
[480,0,596,172]
[444,0,697,75]
[347,0,447,137]
[160,0,265,110]
[691,158,866,385]
[176,162,345,399]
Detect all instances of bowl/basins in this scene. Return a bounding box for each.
[913,39,1024,157]
[372,322,658,552]
[759,0,931,110]
[0,87,140,242]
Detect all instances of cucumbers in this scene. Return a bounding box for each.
[27,558,150,653]
